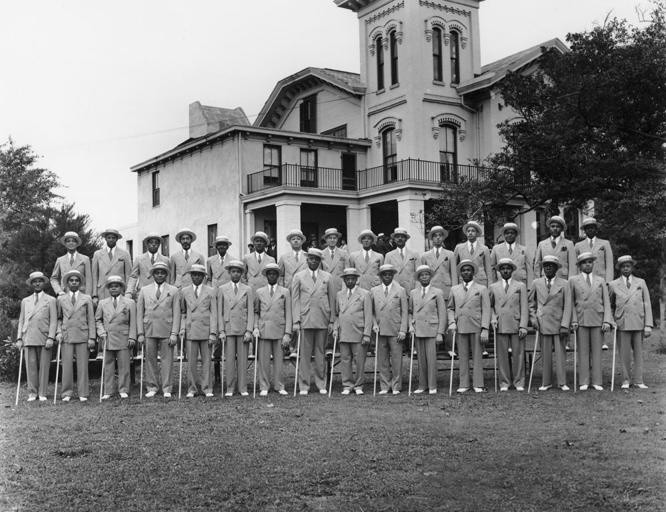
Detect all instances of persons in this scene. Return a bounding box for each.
[331,268,371,396]
[253,263,292,396]
[94,275,136,402]
[568,251,611,391]
[406,265,446,396]
[216,261,254,397]
[609,255,653,389]
[487,259,529,391]
[135,263,179,399]
[351,213,615,358]
[56,270,94,402]
[528,255,571,391]
[49,228,351,361]
[369,264,406,394]
[179,264,217,398]
[446,259,490,392]
[291,247,335,397]
[15,271,56,403]
